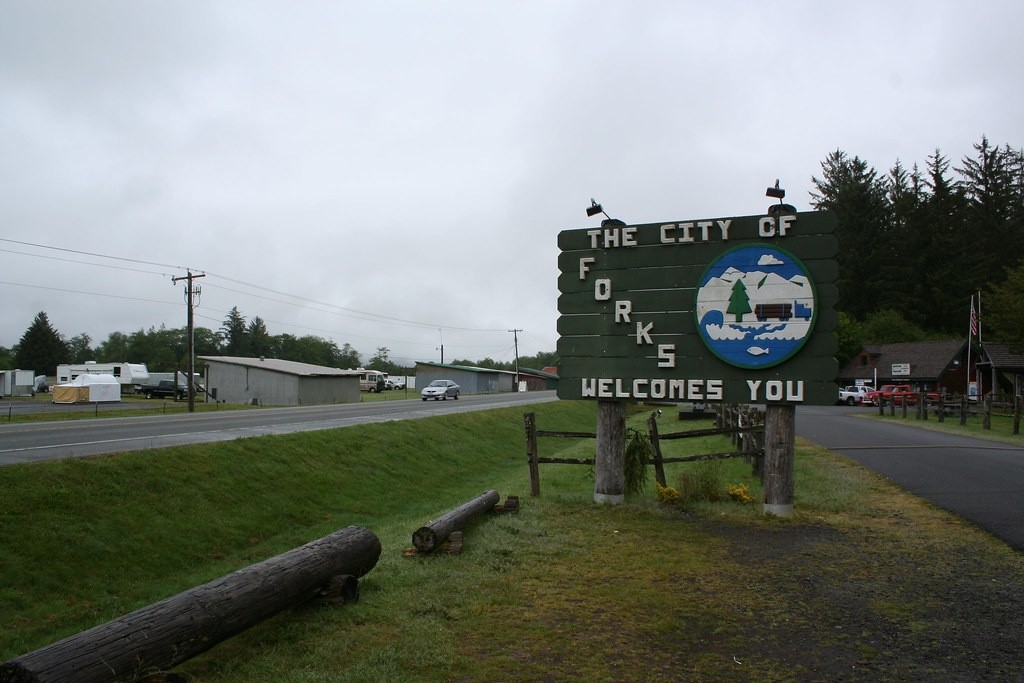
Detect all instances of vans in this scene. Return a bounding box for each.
[359,370,386,394]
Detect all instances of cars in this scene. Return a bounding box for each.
[421,380,461,402]
[384,377,405,391]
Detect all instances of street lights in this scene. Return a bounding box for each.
[438,328,443,365]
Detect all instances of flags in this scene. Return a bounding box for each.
[971,306,979,335]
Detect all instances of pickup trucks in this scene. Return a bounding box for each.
[862,384,941,407]
[838,385,878,407]
[142,379,197,401]
